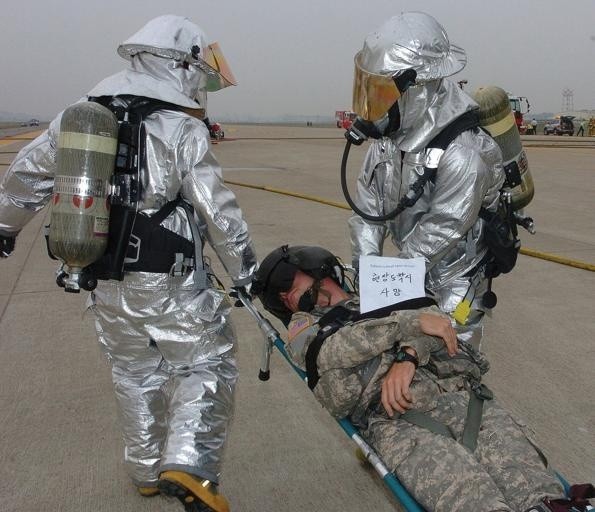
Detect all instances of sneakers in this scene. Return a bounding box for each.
[138,470,230,512]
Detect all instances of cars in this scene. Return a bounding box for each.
[29,118,40,127]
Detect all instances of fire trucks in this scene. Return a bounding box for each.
[334,110,356,131]
[507,92,533,137]
[209,122,226,146]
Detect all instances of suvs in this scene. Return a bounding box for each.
[542,114,577,137]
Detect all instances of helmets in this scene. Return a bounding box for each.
[117,15,237,93]
[257,243,345,320]
[353,10,466,122]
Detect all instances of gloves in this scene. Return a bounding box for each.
[0,234,16,257]
[229,280,257,307]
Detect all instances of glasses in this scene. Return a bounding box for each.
[265,259,296,311]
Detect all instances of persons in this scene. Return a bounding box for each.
[576,120,586,137]
[1,12,262,512]
[341,9,536,359]
[530,118,538,136]
[254,240,595,511]
